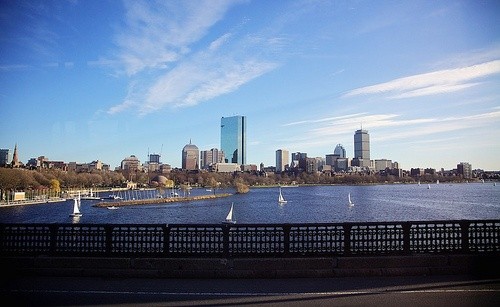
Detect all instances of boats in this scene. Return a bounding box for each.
[75,188,101,201]
[108,194,122,200]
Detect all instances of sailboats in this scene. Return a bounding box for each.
[347,193,354,208]
[69,199,82,218]
[223,203,237,225]
[278,187,287,205]
